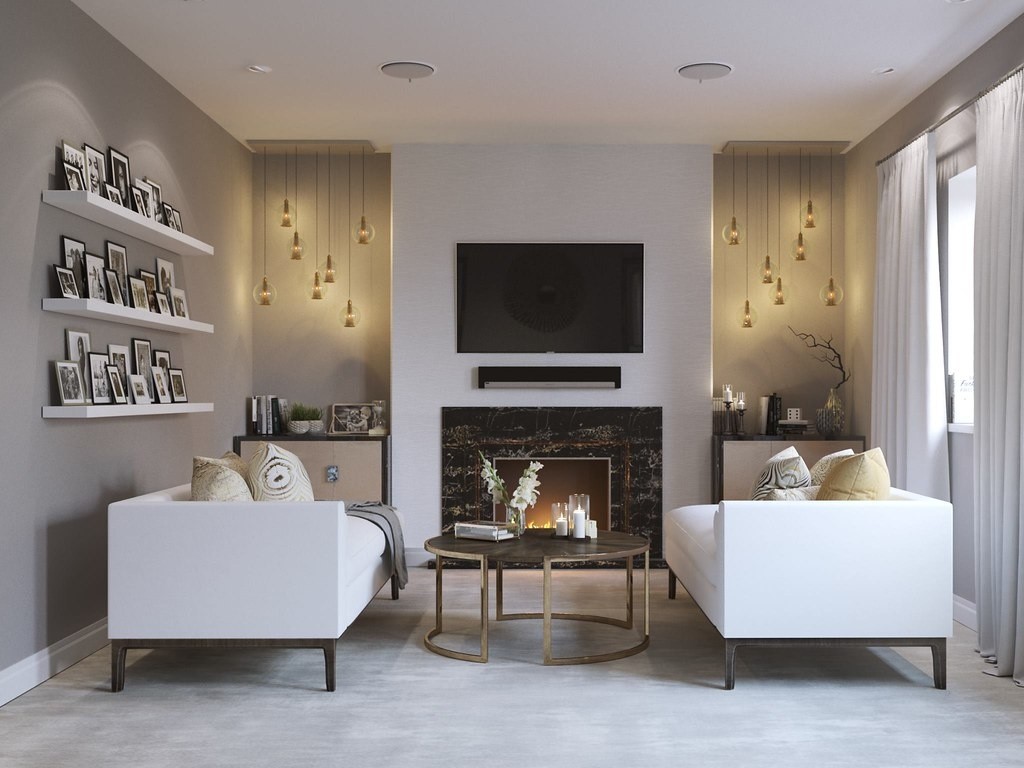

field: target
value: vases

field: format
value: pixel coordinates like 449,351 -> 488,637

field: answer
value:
505,503 -> 526,535
825,388 -> 845,437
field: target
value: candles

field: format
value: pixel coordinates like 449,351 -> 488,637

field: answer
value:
551,493 -> 591,542
737,399 -> 745,410
723,387 -> 732,401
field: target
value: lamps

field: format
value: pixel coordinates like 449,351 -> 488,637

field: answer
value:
721,141 -> 850,327
247,140 -> 375,327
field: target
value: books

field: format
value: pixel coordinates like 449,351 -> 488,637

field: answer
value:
251,395 -> 288,435
776,419 -> 819,434
758,392 -> 782,435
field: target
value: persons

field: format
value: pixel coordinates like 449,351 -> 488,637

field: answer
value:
348,406 -> 371,431
66,151 -> 181,232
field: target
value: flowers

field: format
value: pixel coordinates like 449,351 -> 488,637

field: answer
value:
478,449 -> 544,531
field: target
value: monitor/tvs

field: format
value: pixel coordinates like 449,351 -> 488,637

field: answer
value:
457,239 -> 644,353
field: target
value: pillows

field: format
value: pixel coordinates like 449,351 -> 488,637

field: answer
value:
191,440 -> 314,502
750,445 -> 890,500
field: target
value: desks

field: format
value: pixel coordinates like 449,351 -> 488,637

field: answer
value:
424,528 -> 650,666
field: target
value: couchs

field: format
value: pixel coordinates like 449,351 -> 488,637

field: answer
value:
108,484 -> 399,692
662,488 -> 953,690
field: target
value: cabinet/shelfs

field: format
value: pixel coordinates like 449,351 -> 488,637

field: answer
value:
42,189 -> 215,419
234,435 -> 391,505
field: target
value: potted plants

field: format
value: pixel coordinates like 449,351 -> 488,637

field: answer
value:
286,402 -> 325,435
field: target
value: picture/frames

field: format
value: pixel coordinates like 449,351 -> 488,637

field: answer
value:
332,404 -> 377,433
54,329 -> 189,406
60,139 -> 183,233
54,235 -> 189,320
